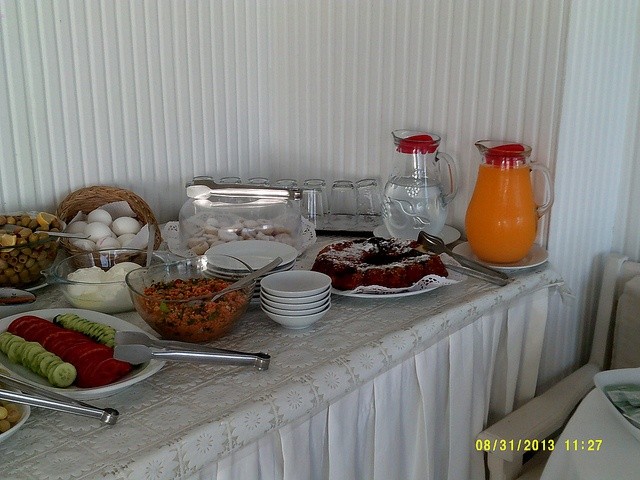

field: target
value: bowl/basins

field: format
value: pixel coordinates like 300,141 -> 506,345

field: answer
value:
259,269 -> 332,329
179,198 -> 302,254
40,248 -> 172,313
125,255 -> 257,344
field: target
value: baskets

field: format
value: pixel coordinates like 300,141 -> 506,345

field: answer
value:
57,186 -> 163,272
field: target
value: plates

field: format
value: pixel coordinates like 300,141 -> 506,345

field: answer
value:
451,241 -> 550,271
331,287 -> 440,299
0,287 -> 37,315
373,224 -> 462,246
0,387 -> 31,443
204,240 -> 298,302
0,307 -> 166,401
25,282 -> 47,292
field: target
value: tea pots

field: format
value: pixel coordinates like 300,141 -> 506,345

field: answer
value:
381,129 -> 458,240
464,140 -> 554,266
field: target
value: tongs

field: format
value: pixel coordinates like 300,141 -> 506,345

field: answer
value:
113,330 -> 271,373
0,374 -> 120,425
184,181 -> 303,200
416,230 -> 509,287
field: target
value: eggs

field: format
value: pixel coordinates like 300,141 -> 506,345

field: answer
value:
111,216 -> 140,236
68,221 -> 86,235
70,239 -> 94,251
117,234 -> 136,243
120,238 -> 135,248
96,238 -> 118,251
87,208 -> 112,225
84,221 -> 110,241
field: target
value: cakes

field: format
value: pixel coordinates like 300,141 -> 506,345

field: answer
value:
308,236 -> 449,290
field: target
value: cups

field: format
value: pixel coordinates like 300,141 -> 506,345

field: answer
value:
193,175 -> 213,180
275,178 -> 298,186
219,176 -> 242,185
301,179 -> 329,224
355,178 -> 381,227
328,179 -> 356,226
247,176 -> 271,186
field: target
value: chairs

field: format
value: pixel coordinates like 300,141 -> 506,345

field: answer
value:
476,253 -> 640,480
